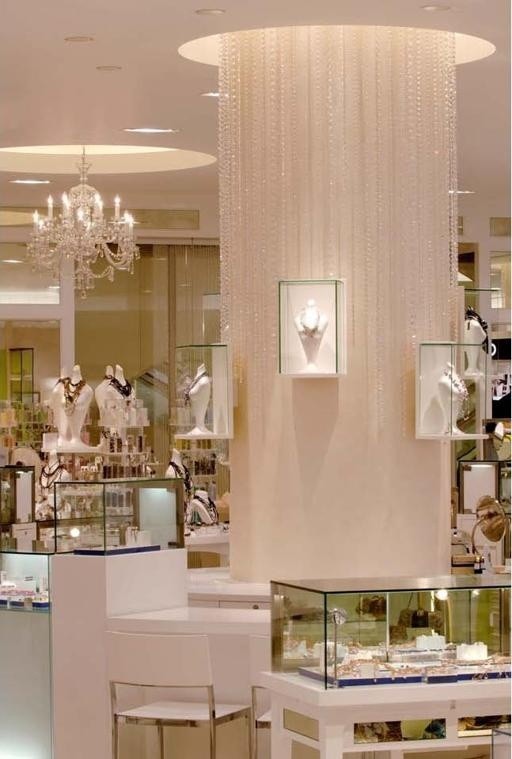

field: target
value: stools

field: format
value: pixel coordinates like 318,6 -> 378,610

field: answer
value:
109,680 -> 252,759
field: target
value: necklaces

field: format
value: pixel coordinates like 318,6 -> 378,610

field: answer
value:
444,369 -> 468,420
300,312 -> 320,337
39,372 -> 219,523
